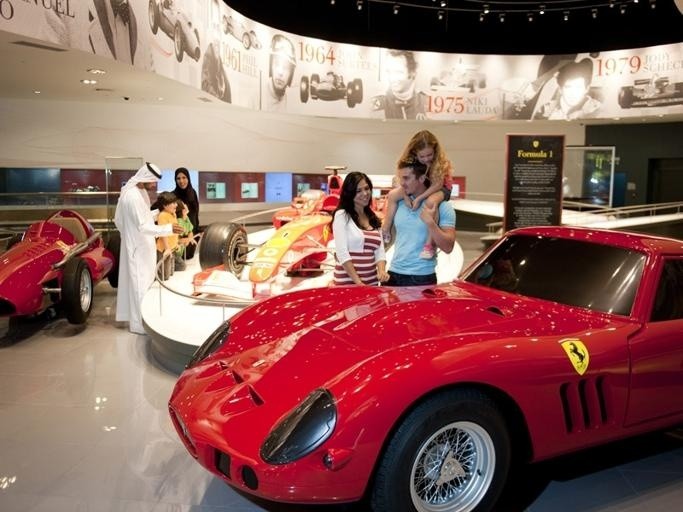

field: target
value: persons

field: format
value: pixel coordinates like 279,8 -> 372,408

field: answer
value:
268,34 -> 296,110
114,161 -> 199,335
383,50 -> 430,120
533,58 -> 602,120
330,129 -> 457,287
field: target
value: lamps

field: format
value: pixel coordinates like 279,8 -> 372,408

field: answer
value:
592,8 -> 597,18
479,13 -> 485,21
538,3 -> 545,14
393,5 -> 399,14
527,12 -> 534,21
610,1 -> 614,8
563,9 -> 569,21
441,1 -> 446,7
483,3 -> 490,13
619,4 -> 627,13
357,2 -> 363,10
331,1 -> 336,5
498,13 -> 505,22
438,11 -> 444,20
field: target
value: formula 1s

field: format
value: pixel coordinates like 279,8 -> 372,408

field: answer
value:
617,76 -> 683,109
191,166 -> 388,301
300,71 -> 363,108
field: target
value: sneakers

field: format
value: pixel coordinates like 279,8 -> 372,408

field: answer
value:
419,244 -> 436,260
382,232 -> 391,243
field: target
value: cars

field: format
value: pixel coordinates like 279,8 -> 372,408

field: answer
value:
0,209 -> 121,346
149,0 -> 200,62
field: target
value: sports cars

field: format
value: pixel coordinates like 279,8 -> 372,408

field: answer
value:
168,225 -> 683,512
